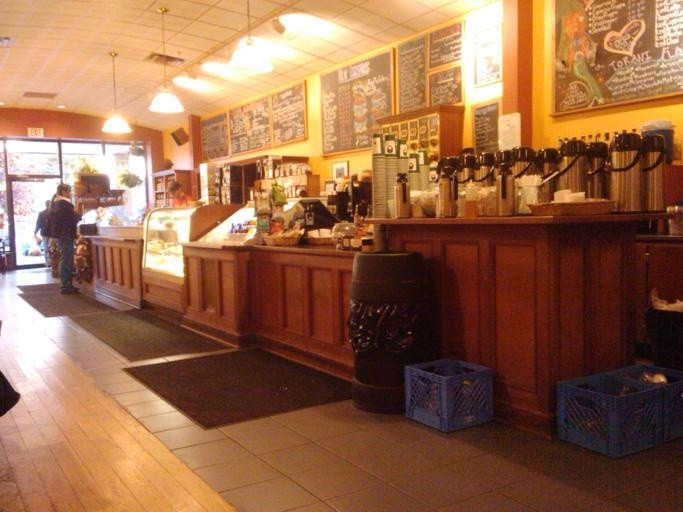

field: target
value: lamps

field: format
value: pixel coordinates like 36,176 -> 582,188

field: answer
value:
226,1 -> 273,79
271,13 -> 287,36
100,52 -> 134,138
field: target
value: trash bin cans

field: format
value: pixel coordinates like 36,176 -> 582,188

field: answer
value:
642,296 -> 683,372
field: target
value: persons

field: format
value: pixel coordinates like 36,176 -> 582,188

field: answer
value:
167,180 -> 193,208
33,200 -> 53,268
48,183 -> 82,295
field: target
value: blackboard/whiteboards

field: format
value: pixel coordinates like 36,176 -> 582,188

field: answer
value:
319,21 -> 464,158
199,80 -> 307,163
550,0 -> 683,118
470,97 -> 503,157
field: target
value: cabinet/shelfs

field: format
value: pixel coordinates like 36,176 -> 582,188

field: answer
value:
150,169 -> 198,208
255,174 -> 320,199
217,155 -> 309,204
373,103 -> 466,167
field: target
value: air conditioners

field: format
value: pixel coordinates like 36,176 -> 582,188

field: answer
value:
148,6 -> 187,117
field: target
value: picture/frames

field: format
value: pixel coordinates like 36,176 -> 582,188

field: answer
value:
330,160 -> 349,183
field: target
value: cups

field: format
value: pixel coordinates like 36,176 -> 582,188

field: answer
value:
566,192 -> 585,202
336,233 -> 372,253
370,133 -> 385,252
428,163 -> 437,192
464,187 -> 476,217
418,150 -> 427,191
665,205 -> 683,236
319,228 -> 333,238
397,139 -> 408,176
553,188 -> 571,202
306,230 -> 318,238
384,135 -> 396,218
408,152 -> 419,191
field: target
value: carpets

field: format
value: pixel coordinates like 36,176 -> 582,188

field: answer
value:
114,347 -> 353,431
15,281 -> 121,318
68,307 -> 233,363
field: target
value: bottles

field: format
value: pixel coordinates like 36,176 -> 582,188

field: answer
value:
396,173 -> 410,219
558,127 -> 637,169
559,12 -> 602,110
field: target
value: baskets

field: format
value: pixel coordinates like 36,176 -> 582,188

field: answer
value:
403,358 -> 494,433
556,363 -> 682,459
261,233 -> 301,246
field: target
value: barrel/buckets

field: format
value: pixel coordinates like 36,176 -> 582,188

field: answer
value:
346,250 -> 437,415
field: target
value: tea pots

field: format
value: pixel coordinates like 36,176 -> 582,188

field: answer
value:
517,185 -> 543,216
331,220 -> 358,246
